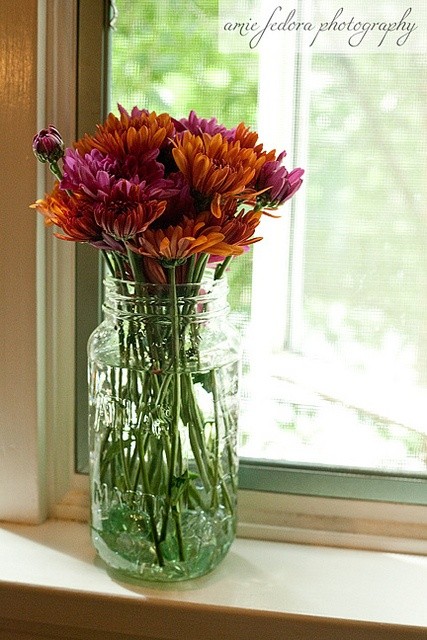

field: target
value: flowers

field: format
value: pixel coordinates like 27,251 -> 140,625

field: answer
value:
83,266 -> 244,591
25,100 -> 309,565
